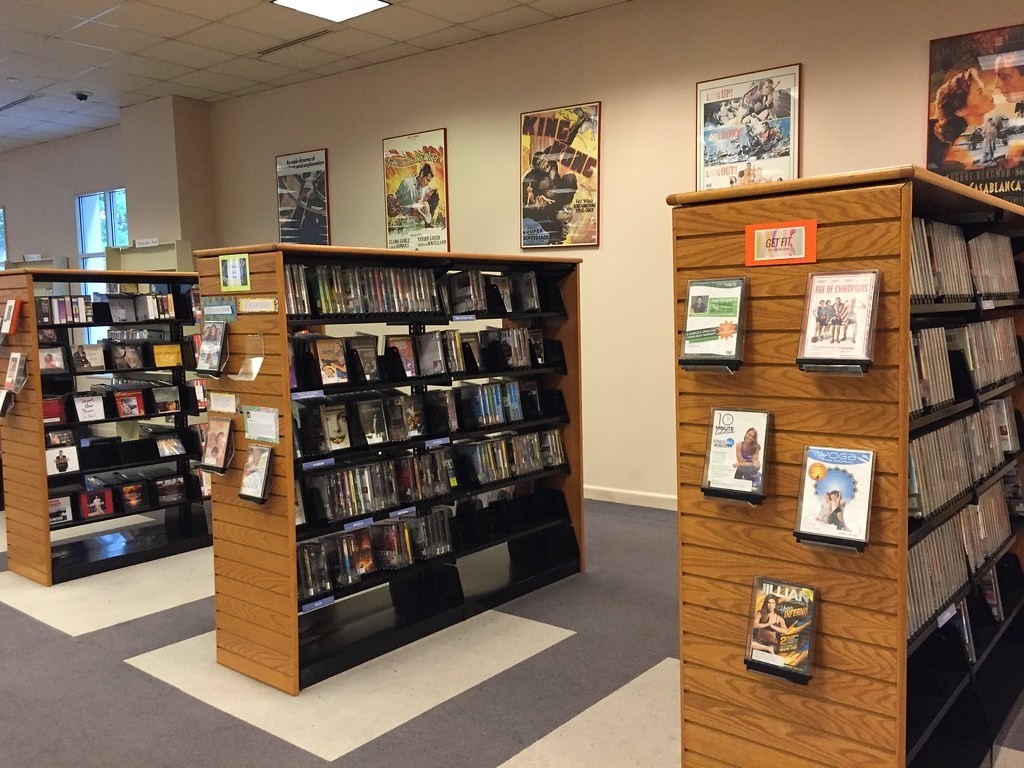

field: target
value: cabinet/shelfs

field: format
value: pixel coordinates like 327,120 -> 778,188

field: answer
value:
0,268 -> 213,588
666,165 -> 1023,768
4,256 -> 69,297
194,243 -> 587,697
105,240 -> 194,296
